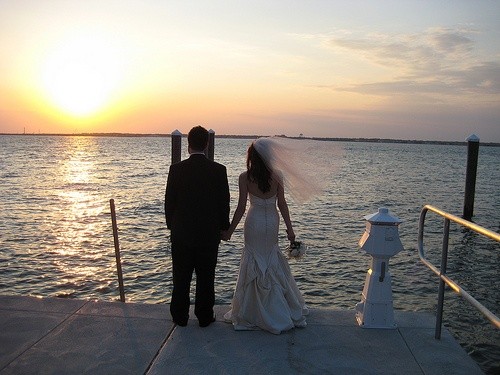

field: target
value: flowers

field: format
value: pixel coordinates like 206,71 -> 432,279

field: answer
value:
286,230 -> 306,260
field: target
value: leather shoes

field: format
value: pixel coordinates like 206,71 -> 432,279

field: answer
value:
173,317 -> 188,327
198,317 -> 216,327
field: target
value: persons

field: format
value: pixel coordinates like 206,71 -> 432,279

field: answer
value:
165,125 -> 232,327
223,137 -> 296,335
